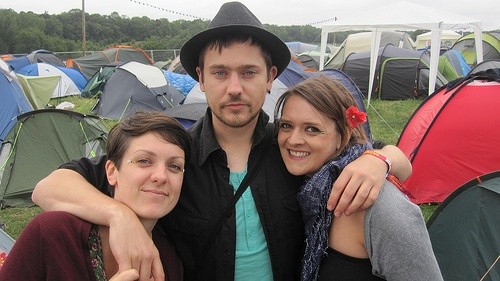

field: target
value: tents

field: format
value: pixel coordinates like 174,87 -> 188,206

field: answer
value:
318,0 -> 483,109
0,30 -> 500,281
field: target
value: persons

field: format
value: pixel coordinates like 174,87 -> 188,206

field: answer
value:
0,111 -> 192,281
31,2 -> 413,281
273,75 -> 443,281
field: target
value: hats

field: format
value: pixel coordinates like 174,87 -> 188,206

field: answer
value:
180,1 -> 292,83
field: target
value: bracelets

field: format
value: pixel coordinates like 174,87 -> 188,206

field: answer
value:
362,150 -> 392,174
